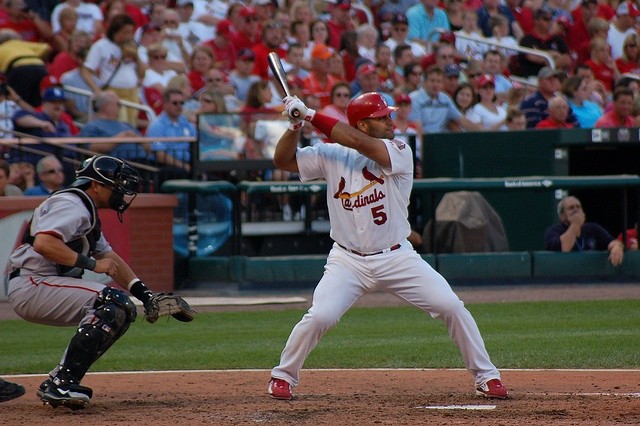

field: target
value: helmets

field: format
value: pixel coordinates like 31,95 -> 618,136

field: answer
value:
347,93 -> 399,126
71,156 -> 140,223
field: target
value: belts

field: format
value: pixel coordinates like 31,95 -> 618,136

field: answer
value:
338,243 -> 401,257
9,267 -> 19,280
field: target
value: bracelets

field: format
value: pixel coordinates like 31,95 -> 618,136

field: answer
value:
76,254 -> 96,270
311,113 -> 339,138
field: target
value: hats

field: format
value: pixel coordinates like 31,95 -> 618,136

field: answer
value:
240,8 -> 256,17
393,14 -> 408,25
238,49 -> 254,61
538,66 -> 568,79
360,65 -> 377,74
45,88 -> 65,102
444,64 -> 459,77
312,45 -> 334,60
480,75 -> 495,86
216,20 -> 238,40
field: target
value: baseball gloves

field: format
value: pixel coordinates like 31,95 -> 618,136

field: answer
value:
144,292 -> 198,324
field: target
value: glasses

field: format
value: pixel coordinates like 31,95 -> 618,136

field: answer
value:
208,78 -> 221,82
47,169 -> 65,172
568,205 -> 581,209
153,55 -> 166,59
205,99 -> 214,103
172,101 -> 184,105
411,72 -> 423,76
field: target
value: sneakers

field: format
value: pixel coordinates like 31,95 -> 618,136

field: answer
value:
476,379 -> 507,399
41,377 -> 92,411
37,379 -> 53,395
268,377 -> 292,399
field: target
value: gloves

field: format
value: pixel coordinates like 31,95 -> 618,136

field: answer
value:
282,96 -> 316,131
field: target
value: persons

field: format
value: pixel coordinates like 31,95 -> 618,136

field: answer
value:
391,1 -> 639,179
545,196 -> 623,267
4,155 -> 196,411
293,1 -> 390,219
270,91 -> 508,400
1,0 -> 292,221
619,228 -> 638,248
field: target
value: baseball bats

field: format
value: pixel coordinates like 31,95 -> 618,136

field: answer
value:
267,52 -> 300,118
